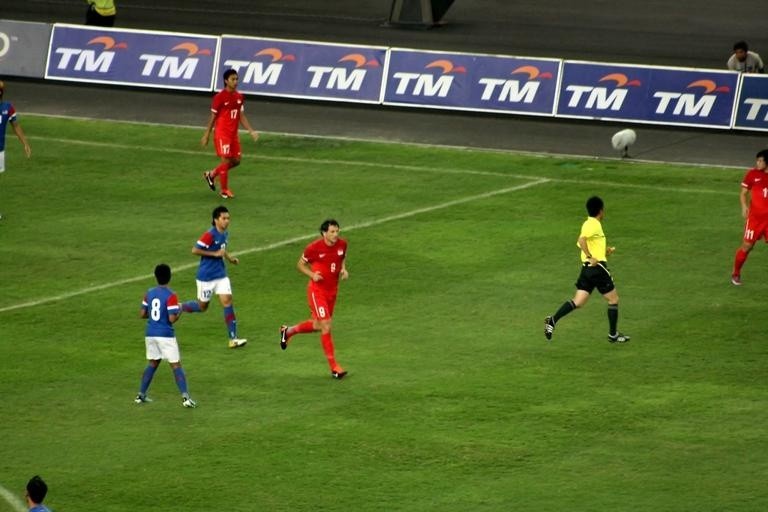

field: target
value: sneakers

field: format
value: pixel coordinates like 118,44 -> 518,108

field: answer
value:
331,365 -> 348,380
280,324 -> 289,350
182,397 -> 199,408
228,337 -> 247,349
134,393 -> 154,404
608,331 -> 630,344
201,171 -> 217,192
544,316 -> 555,341
218,189 -> 237,200
731,273 -> 743,289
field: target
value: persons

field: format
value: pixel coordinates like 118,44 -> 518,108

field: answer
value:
176,206 -> 247,348
726,41 -> 764,74
133,264 -> 199,408
279,219 -> 348,379
26,476 -> 55,512
544,196 -> 631,342
731,150 -> 768,286
201,69 -> 261,199
0,80 -> 31,173
85,0 -> 116,27
